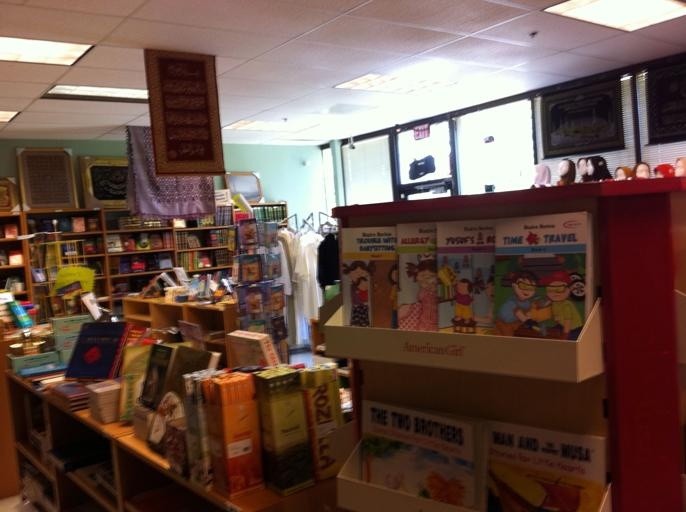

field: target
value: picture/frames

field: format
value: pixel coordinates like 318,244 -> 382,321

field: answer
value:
0,146 -> 131,213
222,170 -> 266,205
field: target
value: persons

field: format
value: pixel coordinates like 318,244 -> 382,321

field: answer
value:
529,155 -> 686,190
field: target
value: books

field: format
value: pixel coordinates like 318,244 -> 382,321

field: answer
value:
337,209 -> 606,512
30,207 -> 286,460
